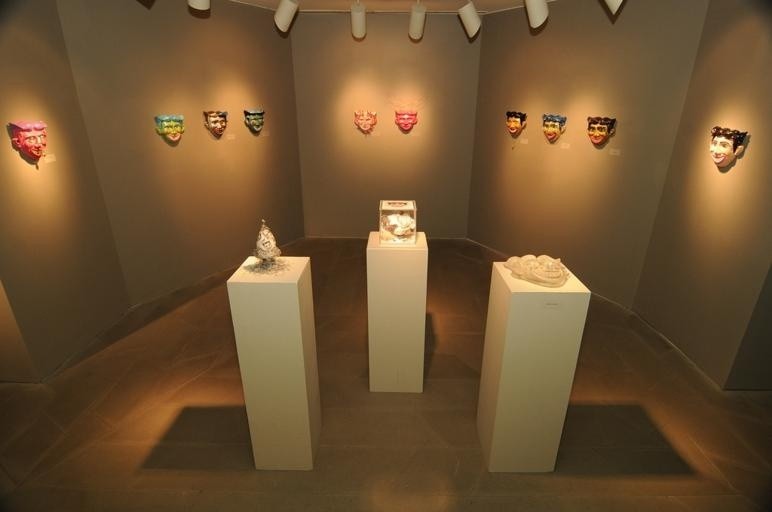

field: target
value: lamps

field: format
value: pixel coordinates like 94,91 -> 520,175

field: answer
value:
187,1 -> 211,12
603,0 -> 625,16
523,0 -> 551,34
272,0 -> 298,34
408,0 -> 429,41
458,1 -> 483,39
349,0 -> 370,39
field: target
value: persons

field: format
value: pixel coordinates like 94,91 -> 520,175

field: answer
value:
708,126 -> 748,169
202,110 -> 228,138
154,113 -> 185,146
586,116 -> 617,145
543,113 -> 566,144
506,111 -> 526,136
394,110 -> 418,132
8,120 -> 48,160
353,111 -> 377,133
243,110 -> 265,133
